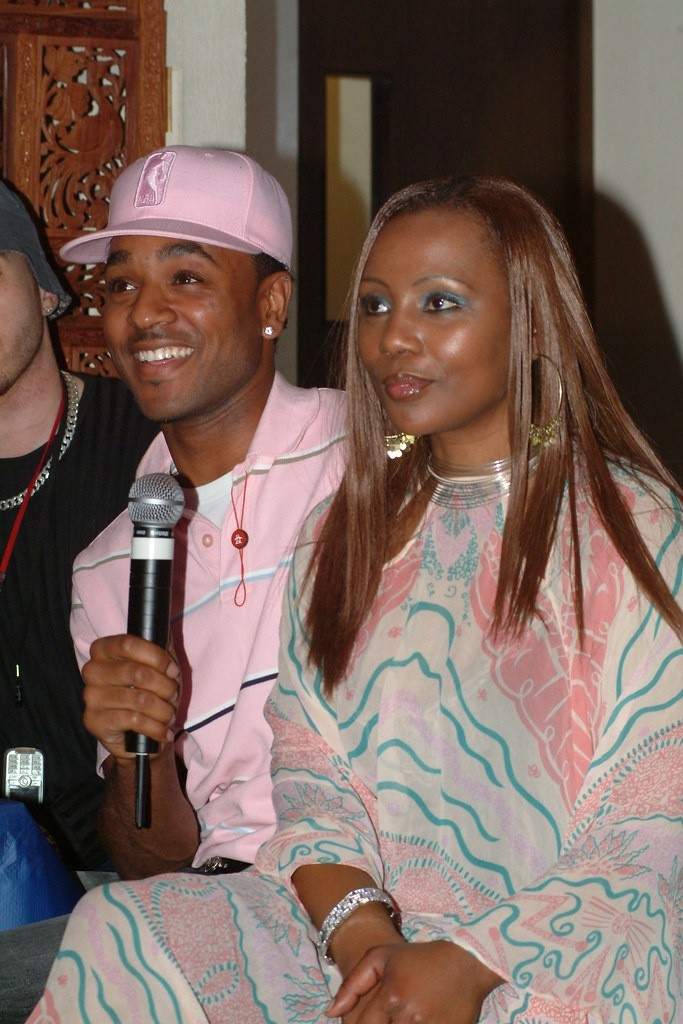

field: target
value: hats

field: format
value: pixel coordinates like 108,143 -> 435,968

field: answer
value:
57,144 -> 294,279
0,178 -> 72,320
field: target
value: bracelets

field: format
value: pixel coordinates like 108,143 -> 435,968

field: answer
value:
317,887 -> 398,966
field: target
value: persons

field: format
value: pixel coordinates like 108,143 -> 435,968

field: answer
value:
25,177 -> 683,1024
0,144 -> 347,1024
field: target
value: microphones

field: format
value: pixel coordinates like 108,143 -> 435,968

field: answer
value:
127,471 -> 185,831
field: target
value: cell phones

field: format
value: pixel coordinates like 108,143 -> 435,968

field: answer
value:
5,748 -> 44,805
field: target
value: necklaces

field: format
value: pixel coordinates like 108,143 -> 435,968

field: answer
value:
418,446 -> 539,509
0,369 -> 78,511
